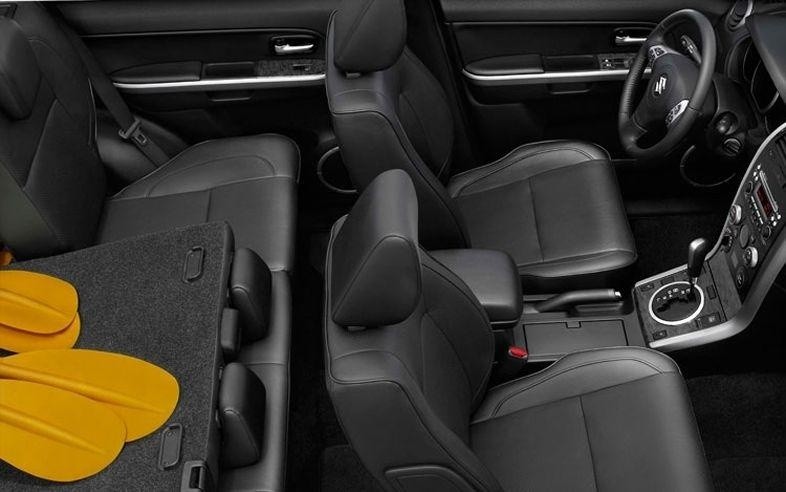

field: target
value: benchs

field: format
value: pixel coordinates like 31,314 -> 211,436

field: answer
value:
1,0 -> 322,491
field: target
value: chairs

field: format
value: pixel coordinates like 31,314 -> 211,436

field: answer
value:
324,170 -> 714,492
327,3 -> 640,295
